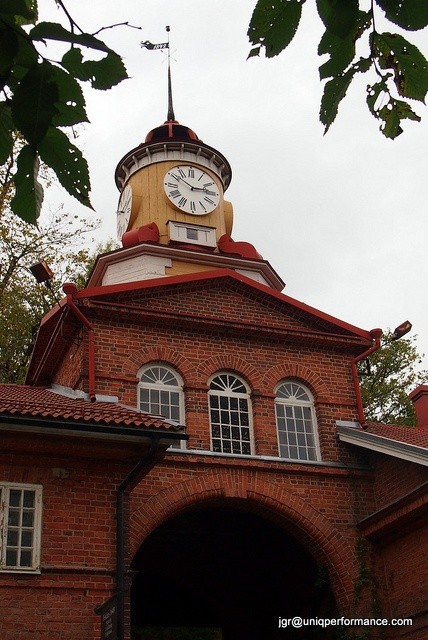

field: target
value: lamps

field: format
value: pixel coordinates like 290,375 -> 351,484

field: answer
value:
383,320 -> 412,346
28,260 -> 69,325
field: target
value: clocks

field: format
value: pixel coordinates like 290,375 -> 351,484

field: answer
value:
163,165 -> 221,216
116,184 -> 133,242
